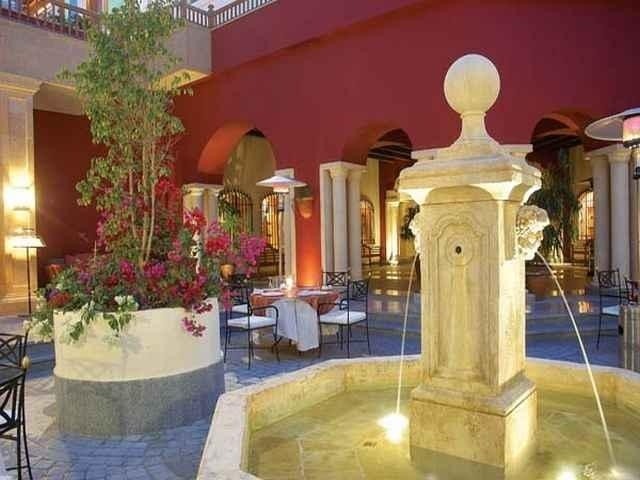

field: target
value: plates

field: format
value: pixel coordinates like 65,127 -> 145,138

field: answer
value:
262,292 -> 282,297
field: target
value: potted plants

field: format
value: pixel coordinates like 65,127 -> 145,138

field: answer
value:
526,142 -> 581,296
401,204 -> 420,280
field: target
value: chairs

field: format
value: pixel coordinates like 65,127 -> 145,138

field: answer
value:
257,242 -> 285,277
326,271 -> 353,339
224,286 -> 280,370
361,238 -> 381,266
226,274 -> 262,344
596,268 -> 629,349
316,273 -> 372,359
624,276 -> 640,306
0,328 -> 34,480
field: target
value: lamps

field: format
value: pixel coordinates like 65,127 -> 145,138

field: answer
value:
256,175 -> 307,284
9,227 -> 46,317
584,105 -> 640,279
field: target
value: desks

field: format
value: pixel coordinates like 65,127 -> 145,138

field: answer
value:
251,288 -> 339,353
